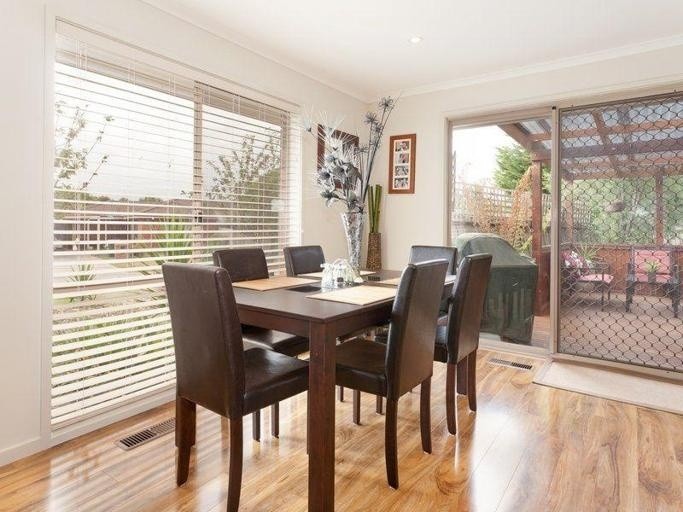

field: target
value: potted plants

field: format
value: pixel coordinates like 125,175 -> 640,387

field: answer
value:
574,243 -> 600,291
642,260 -> 661,286
290,88 -> 409,276
366,184 -> 382,270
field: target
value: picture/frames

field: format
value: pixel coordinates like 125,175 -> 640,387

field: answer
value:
388,134 -> 416,195
317,124 -> 360,191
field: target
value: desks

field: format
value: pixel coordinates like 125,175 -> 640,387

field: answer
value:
174,269 -> 458,512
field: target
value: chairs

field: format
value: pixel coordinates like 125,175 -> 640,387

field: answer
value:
546,243 -> 615,313
283,245 -> 371,401
373,245 -> 458,394
335,258 -> 449,490
212,246 -> 309,442
161,262 -> 309,511
374,253 -> 493,436
625,242 -> 681,318
